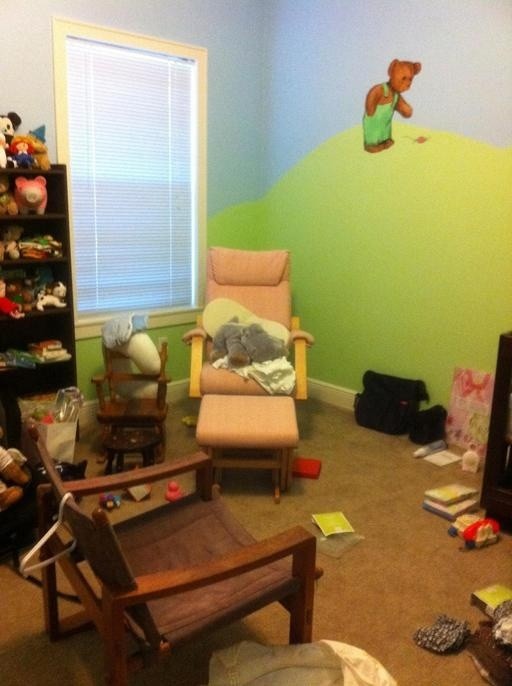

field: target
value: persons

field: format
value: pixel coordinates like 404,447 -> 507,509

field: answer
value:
0,443 -> 32,513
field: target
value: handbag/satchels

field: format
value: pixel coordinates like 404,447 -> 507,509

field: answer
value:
354,370 -> 428,435
409,405 -> 446,445
21,400 -> 77,470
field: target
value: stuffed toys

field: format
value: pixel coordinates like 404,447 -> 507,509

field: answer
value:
0,110 -> 67,321
200,296 -> 291,352
208,313 -> 287,368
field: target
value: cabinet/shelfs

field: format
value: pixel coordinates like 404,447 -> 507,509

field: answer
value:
482,331 -> 512,535
1,163 -> 80,443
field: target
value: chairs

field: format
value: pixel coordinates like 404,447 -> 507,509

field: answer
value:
184,247 -> 315,416
91,341 -> 171,464
31,441 -> 324,686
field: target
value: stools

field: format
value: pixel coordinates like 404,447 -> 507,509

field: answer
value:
197,393 -> 299,504
98,431 -> 160,475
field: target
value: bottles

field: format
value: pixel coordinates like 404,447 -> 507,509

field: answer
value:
462,443 -> 481,473
412,439 -> 447,459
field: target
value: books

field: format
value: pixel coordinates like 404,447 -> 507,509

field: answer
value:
423,482 -> 480,507
0,338 -> 73,369
470,584 -> 512,618
423,497 -> 481,520
311,509 -> 355,538
